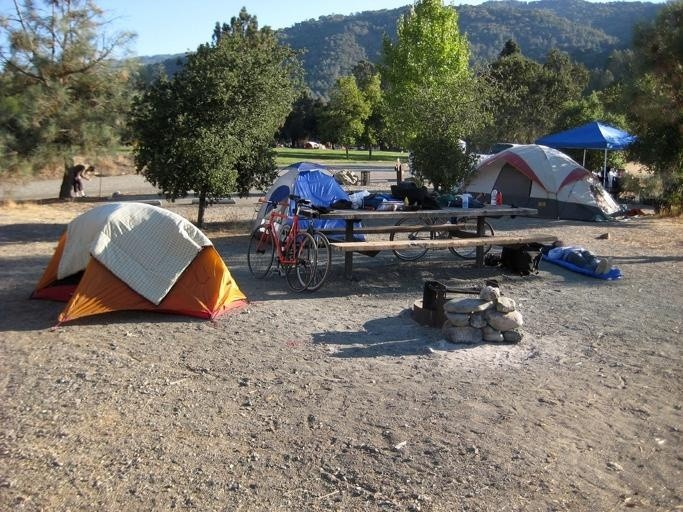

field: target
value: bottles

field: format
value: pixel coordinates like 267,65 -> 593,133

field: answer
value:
496,191 -> 502,207
491,188 -> 497,206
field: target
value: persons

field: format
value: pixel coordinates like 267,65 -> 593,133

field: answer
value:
592,165 -> 625,190
346,190 -> 409,211
547,240 -> 613,276
69,162 -> 96,198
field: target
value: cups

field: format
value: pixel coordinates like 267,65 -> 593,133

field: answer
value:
462,195 -> 468,209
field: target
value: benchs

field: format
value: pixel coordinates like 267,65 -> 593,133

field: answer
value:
299,223 -> 465,234
330,234 -> 558,280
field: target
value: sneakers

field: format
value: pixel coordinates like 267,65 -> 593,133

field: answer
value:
603,257 -> 613,274
593,258 -> 608,275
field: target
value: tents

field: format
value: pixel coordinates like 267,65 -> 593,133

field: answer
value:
252,160 -> 365,243
456,142 -> 628,223
30,201 -> 250,331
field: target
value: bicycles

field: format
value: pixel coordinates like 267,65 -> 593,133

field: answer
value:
282,194 -> 331,291
389,200 -> 494,262
247,196 -> 317,294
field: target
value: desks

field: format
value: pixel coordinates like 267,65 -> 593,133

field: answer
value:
320,208 -> 539,240
